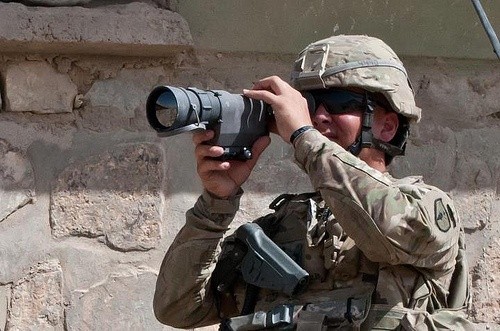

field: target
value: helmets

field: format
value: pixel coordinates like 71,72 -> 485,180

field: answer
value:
292,34 -> 421,120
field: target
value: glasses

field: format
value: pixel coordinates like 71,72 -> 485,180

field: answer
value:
302,89 -> 388,115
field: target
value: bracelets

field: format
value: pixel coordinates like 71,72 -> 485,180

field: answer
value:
290,126 -> 315,145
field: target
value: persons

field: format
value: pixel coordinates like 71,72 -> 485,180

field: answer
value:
153,35 -> 471,331
